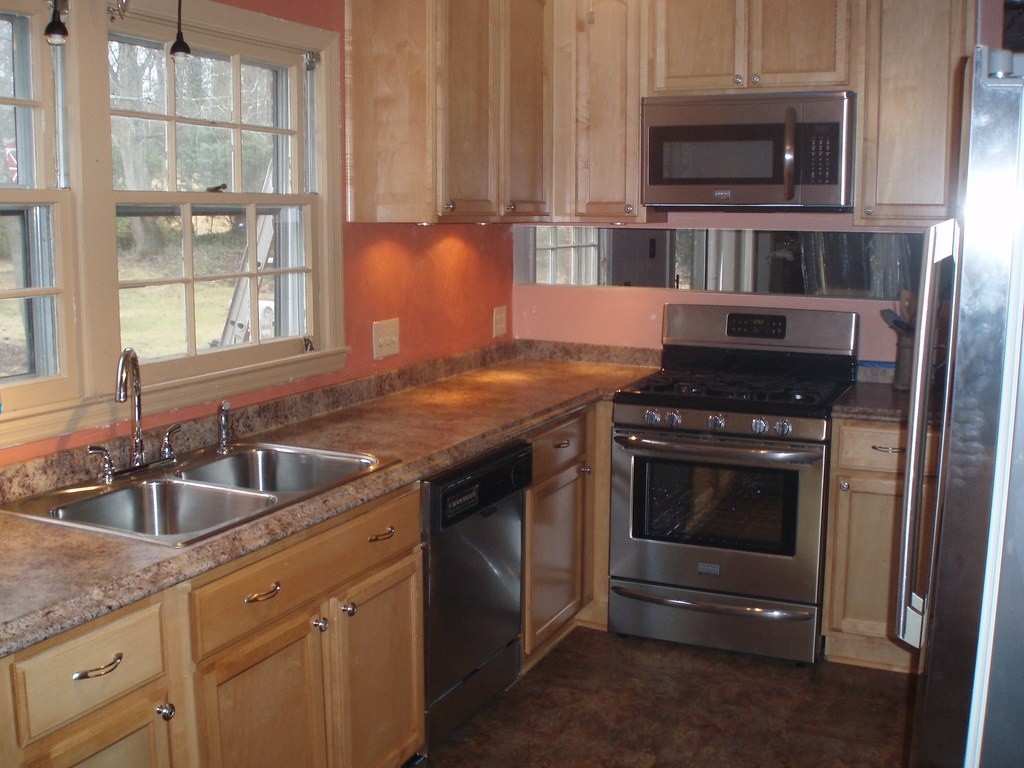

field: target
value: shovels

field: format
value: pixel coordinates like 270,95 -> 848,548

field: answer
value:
900,289 -> 917,324
881,309 -> 900,328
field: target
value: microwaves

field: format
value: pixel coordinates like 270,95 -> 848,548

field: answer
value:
638,89 -> 858,212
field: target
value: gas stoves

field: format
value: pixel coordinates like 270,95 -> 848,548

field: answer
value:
612,303 -> 860,420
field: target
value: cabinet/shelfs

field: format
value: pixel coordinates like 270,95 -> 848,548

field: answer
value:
522,400 -> 595,676
850,0 -> 976,226
1,480 -> 425,768
822,415 -> 945,676
644,0 -> 850,93
545,0 -> 644,227
344,0 -> 552,223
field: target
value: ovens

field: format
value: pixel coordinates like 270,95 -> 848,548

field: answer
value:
609,403 -> 827,666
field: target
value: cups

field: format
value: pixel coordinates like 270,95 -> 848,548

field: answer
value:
893,334 -> 915,391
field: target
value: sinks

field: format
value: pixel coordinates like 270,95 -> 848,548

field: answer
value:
142,442 -> 400,500
0,469 -> 296,546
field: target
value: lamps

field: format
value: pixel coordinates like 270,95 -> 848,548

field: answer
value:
169,0 -> 190,60
44,0 -> 69,46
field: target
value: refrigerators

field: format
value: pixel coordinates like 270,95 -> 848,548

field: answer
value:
892,43 -> 1024,768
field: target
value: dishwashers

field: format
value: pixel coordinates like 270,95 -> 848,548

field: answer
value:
419,439 -> 526,759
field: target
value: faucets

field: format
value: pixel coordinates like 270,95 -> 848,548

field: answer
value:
215,399 -> 232,455
87,347 -> 182,485
